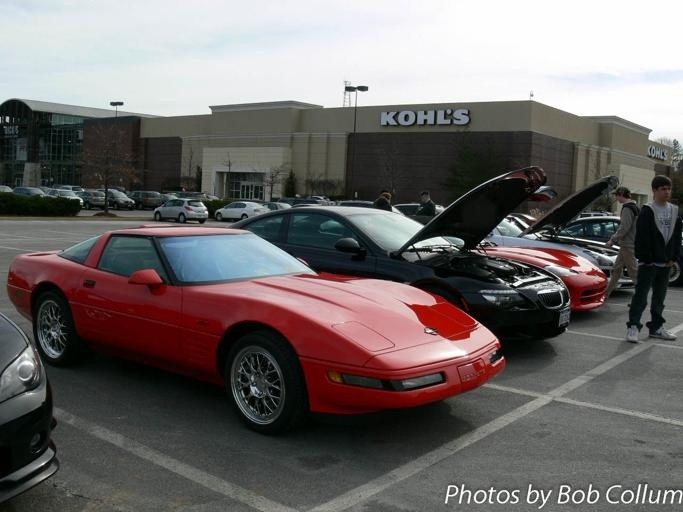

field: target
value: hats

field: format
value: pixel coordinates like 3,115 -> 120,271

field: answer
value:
610,187 -> 631,197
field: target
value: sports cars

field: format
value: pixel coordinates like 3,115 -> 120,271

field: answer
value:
440,230 -> 611,314
226,166 -> 573,349
4,221 -> 509,437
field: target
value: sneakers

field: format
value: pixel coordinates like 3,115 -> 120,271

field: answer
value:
626,325 -> 639,342
649,325 -> 677,339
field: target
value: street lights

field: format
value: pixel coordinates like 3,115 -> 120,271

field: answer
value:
106,99 -> 123,121
343,83 -> 368,194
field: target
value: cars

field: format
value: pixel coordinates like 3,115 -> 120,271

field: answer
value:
0,311 -> 61,504
151,197 -> 210,224
212,200 -> 271,221
0,182 -> 224,209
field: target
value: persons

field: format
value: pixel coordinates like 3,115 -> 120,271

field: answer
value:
366,189 -> 393,212
625,174 -> 683,343
604,186 -> 640,307
416,190 -> 435,216
